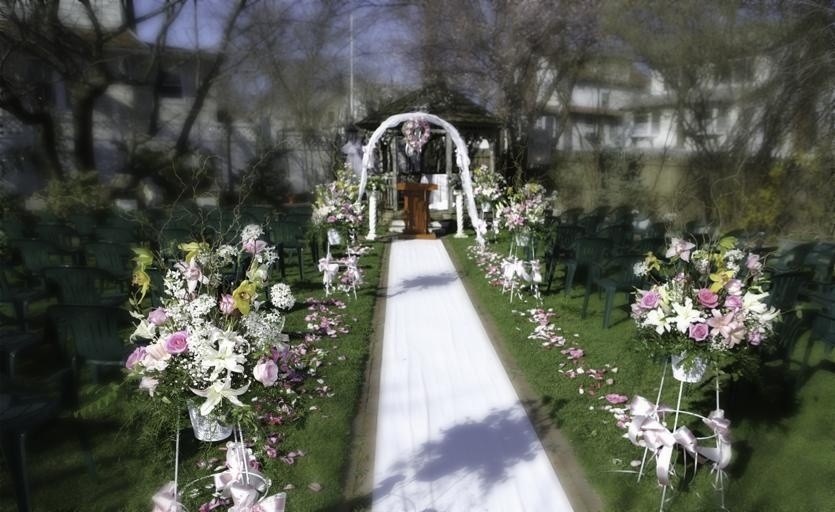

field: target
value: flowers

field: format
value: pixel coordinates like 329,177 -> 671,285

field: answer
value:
469,163 -> 563,239
115,225 -> 297,442
402,119 -> 431,150
308,163 -> 365,229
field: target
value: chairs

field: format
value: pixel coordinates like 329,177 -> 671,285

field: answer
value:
545,205 -> 834,385
0,207 -> 325,512
627,227 -> 785,393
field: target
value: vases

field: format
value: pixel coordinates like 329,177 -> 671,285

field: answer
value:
515,226 -> 531,248
186,393 -> 234,442
327,227 -> 342,245
